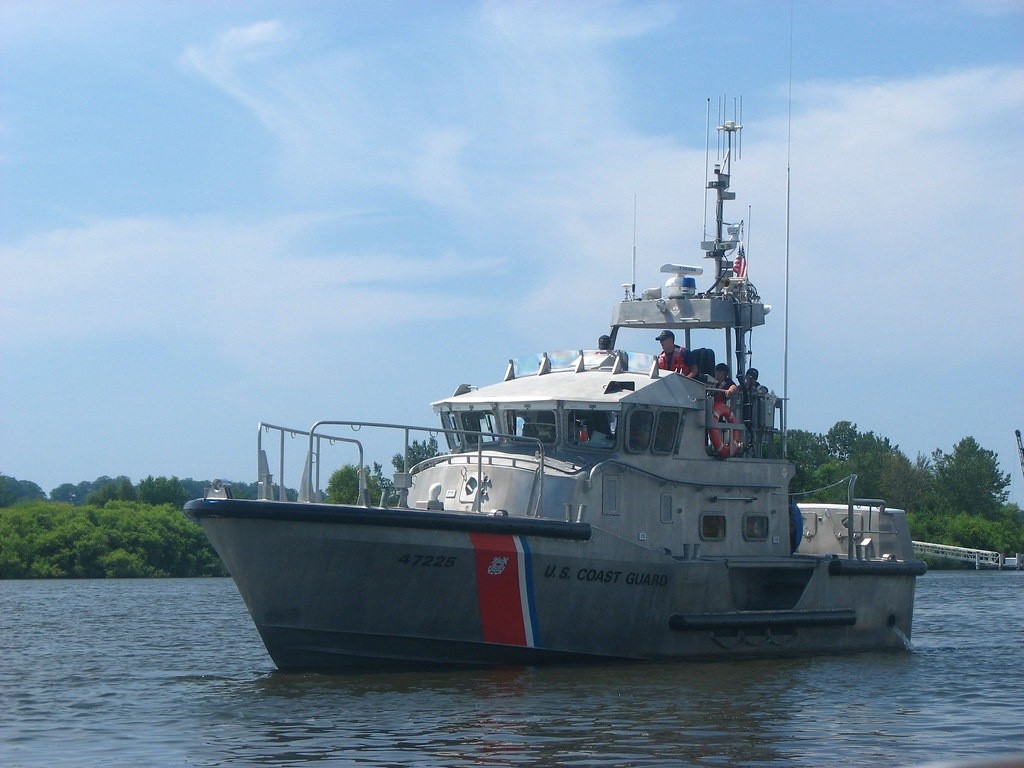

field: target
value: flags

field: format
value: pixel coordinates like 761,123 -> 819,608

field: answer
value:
733,242 -> 747,278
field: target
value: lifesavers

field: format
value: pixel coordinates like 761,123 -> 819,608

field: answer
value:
709,403 -> 739,457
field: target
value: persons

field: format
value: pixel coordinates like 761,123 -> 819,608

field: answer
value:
713,362 -> 738,409
654,330 -> 698,380
598,335 -> 611,350
739,368 -> 768,421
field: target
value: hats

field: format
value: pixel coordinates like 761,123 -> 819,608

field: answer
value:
745,368 -> 758,379
655,330 -> 674,341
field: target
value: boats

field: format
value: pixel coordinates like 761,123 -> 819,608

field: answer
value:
181,93 -> 928,674
